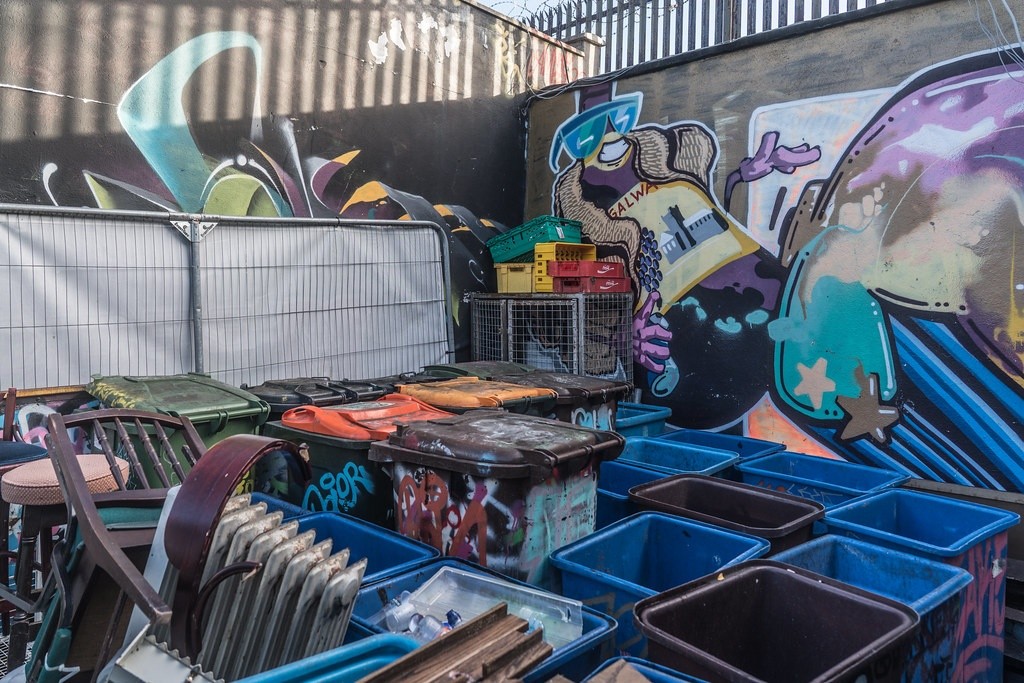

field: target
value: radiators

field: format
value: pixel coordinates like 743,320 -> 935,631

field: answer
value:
146,493 -> 370,683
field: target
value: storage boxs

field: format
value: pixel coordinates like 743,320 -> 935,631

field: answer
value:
483,211 -> 630,294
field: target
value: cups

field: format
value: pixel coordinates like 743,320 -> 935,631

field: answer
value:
384,595 -> 463,646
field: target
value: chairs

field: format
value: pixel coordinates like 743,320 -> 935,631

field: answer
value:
24,409 -> 209,683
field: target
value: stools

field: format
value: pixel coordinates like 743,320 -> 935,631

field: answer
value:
1,451 -> 130,672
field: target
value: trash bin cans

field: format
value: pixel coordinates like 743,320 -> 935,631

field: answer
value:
89,360 -> 998,681
